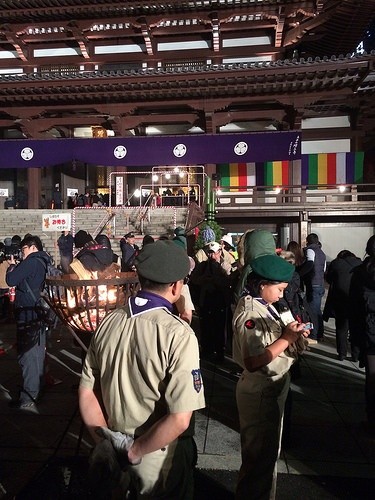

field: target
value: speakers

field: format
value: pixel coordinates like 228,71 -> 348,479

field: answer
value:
212,173 -> 221,180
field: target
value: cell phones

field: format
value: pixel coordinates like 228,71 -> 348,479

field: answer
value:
303,323 -> 314,331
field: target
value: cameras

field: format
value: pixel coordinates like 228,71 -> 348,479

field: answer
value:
5,251 -> 19,259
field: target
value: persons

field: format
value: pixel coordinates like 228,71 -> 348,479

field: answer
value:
141,186 -> 197,206
4,198 -> 10,209
6,234 -> 51,407
15,198 -> 21,208
323,249 -> 364,362
41,196 -> 47,209
67,189 -> 116,210
231,254 -> 310,500
349,235 -> 375,432
77,240 -> 206,500
0,228 -> 326,346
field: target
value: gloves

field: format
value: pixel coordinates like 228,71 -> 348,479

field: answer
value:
91,438 -> 123,468
288,333 -> 310,354
95,427 -> 141,466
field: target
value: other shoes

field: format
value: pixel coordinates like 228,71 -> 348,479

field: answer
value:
307,337 -> 318,345
339,355 -> 346,360
8,398 -> 39,407
352,358 -> 358,362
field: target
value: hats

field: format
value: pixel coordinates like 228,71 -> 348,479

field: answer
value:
250,255 -> 295,283
220,234 -> 234,247
134,240 -> 191,283
74,230 -> 93,248
205,241 -> 223,253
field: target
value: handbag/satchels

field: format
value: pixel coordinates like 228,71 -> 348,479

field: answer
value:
35,297 -> 57,324
